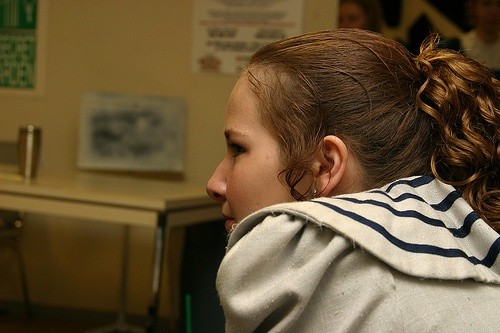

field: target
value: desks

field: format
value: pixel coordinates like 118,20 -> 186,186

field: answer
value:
0,165 -> 227,333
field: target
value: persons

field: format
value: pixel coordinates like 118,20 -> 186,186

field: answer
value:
339,0 -> 380,33
203,31 -> 500,333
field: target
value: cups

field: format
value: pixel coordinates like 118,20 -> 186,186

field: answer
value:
17,125 -> 41,178
